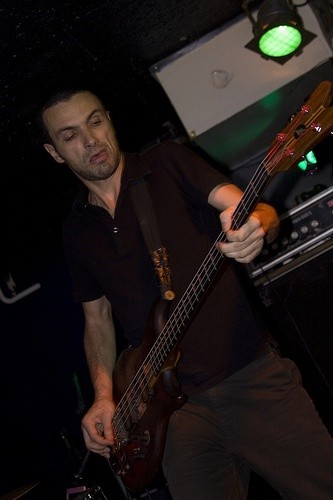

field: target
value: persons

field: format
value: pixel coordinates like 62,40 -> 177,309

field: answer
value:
31,68 -> 333,500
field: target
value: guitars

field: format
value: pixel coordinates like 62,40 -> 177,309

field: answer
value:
108,79 -> 332,487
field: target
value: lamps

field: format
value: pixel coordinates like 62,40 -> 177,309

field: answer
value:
240,1 -> 319,65
